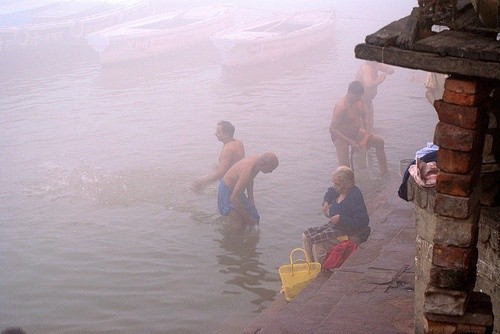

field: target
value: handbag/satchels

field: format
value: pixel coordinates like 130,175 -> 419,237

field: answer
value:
279,248 -> 322,302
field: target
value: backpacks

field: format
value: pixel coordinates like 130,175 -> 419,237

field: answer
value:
321,240 -> 357,272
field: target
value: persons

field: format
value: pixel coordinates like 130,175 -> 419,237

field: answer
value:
218,152 -> 279,237
424,72 -> 450,106
354,60 -> 394,132
302,165 -> 369,262
192,119 -> 246,195
329,81 -> 388,172
